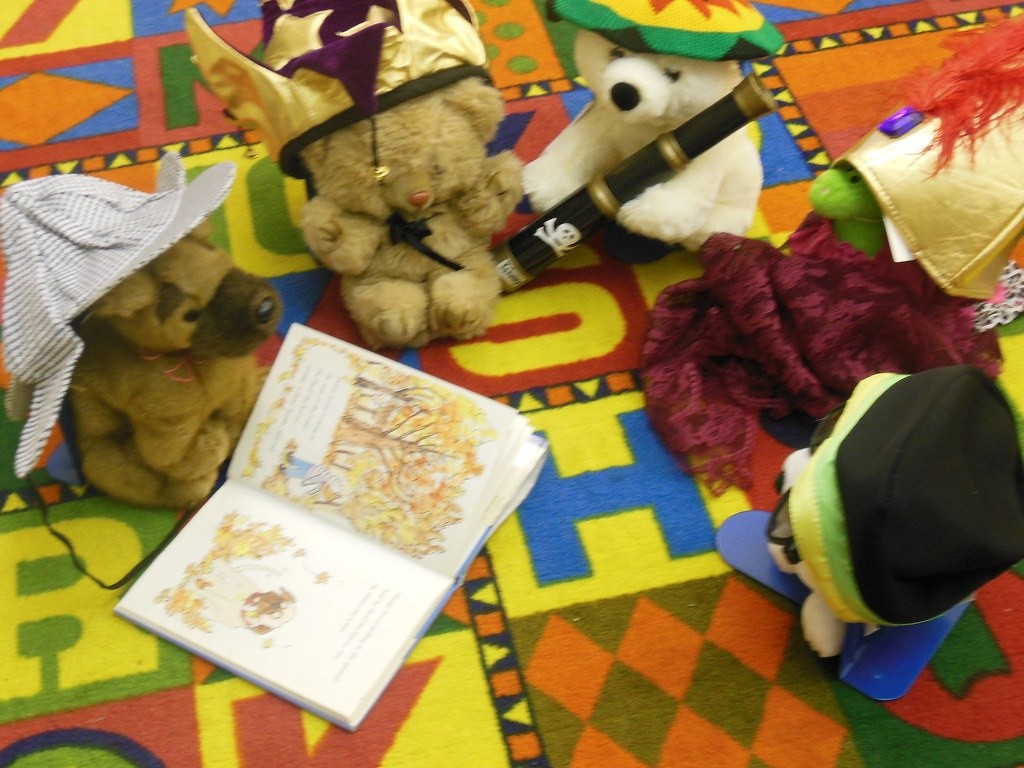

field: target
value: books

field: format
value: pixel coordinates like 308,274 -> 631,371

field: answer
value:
111,322 -> 550,732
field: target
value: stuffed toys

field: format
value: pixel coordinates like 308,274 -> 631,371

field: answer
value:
763,367 -> 1024,661
296,75 -> 526,349
521,30 -> 765,252
811,163 -> 886,261
63,218 -> 284,513
